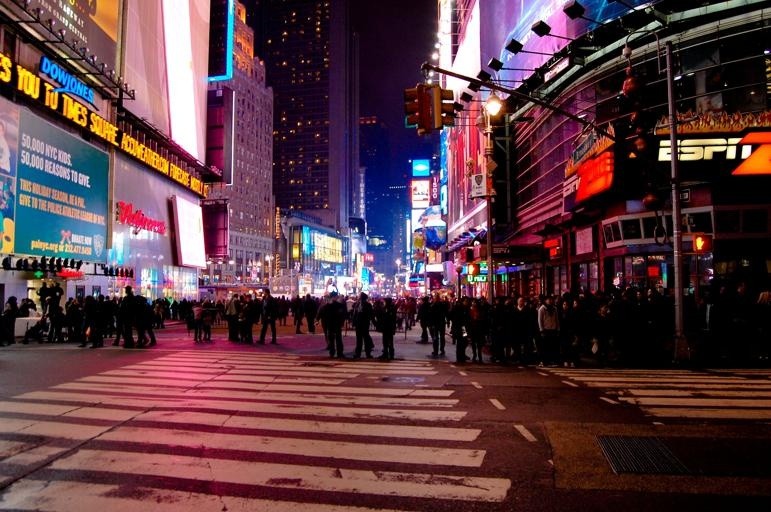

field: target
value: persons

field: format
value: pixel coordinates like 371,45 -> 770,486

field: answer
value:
2,280 -> 771,368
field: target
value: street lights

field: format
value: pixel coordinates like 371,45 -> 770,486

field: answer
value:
396,258 -> 401,280
265,254 -> 275,289
475,96 -> 508,306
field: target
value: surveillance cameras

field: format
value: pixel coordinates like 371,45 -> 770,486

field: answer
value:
622,47 -> 632,59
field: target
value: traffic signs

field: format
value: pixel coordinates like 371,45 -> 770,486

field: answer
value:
679,189 -> 690,202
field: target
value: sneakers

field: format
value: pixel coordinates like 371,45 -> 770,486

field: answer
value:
279,321 -> 316,335
189,333 -> 277,346
0,324 -> 167,349
416,340 -> 428,343
322,343 -> 396,362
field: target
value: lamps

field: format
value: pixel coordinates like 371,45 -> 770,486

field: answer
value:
440,0 -> 639,130
1,248 -> 136,279
1,1 -> 138,105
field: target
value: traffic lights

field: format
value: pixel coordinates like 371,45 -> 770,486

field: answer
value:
692,233 -> 714,254
430,83 -> 455,129
469,265 -> 480,274
630,149 -> 667,212
403,82 -> 432,136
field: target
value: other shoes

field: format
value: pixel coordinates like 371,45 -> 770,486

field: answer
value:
427,348 -> 576,369
